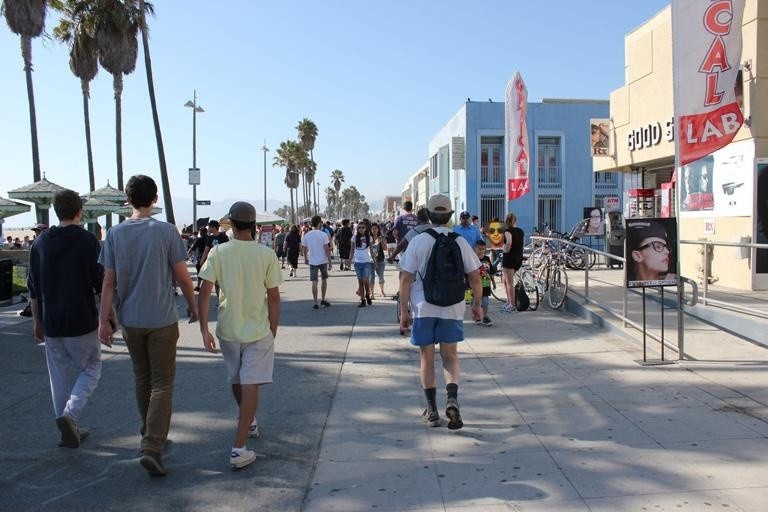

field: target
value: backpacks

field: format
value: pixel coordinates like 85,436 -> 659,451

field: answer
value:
418,228 -> 465,306
515,282 -> 529,311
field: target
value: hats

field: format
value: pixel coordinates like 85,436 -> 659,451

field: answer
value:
427,194 -> 451,214
31,224 -> 48,230
461,212 -> 469,216
628,221 -> 667,240
229,202 -> 256,222
207,220 -> 219,226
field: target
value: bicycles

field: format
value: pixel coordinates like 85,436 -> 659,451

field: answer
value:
513,230 -> 575,314
529,216 -> 594,272
478,252 -> 538,313
393,295 -> 421,330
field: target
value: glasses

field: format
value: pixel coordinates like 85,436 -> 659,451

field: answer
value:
723,183 -> 744,194
358,227 -> 365,228
488,228 -> 504,233
462,217 -> 469,219
638,241 -> 670,252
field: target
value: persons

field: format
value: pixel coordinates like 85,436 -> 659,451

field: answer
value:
454,212 -> 524,311
681,164 -> 699,210
197,200 -> 285,470
591,124 -> 609,148
98,174 -> 200,477
13,238 -> 23,248
397,193 -> 484,430
22,236 -> 31,248
470,239 -> 497,328
3,236 -> 17,249
393,200 -> 420,246
26,188 -> 117,450
583,208 -> 604,235
181,220 -> 233,306
17,223 -> 48,318
628,221 -> 676,281
255,216 -> 393,310
695,161 -> 710,208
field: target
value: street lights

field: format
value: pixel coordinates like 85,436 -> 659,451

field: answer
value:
184,90 -> 203,234
260,137 -> 269,212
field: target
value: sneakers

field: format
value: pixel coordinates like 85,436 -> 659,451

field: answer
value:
141,453 -> 167,475
422,408 -> 440,426
289,268 -> 293,276
312,305 -> 319,310
230,446 -> 256,468
367,296 -> 371,305
446,398 -> 463,429
57,416 -> 89,448
248,416 -> 260,437
320,301 -> 329,306
358,300 -> 366,307
476,317 -> 492,325
500,302 -> 517,313
341,263 -> 351,271
